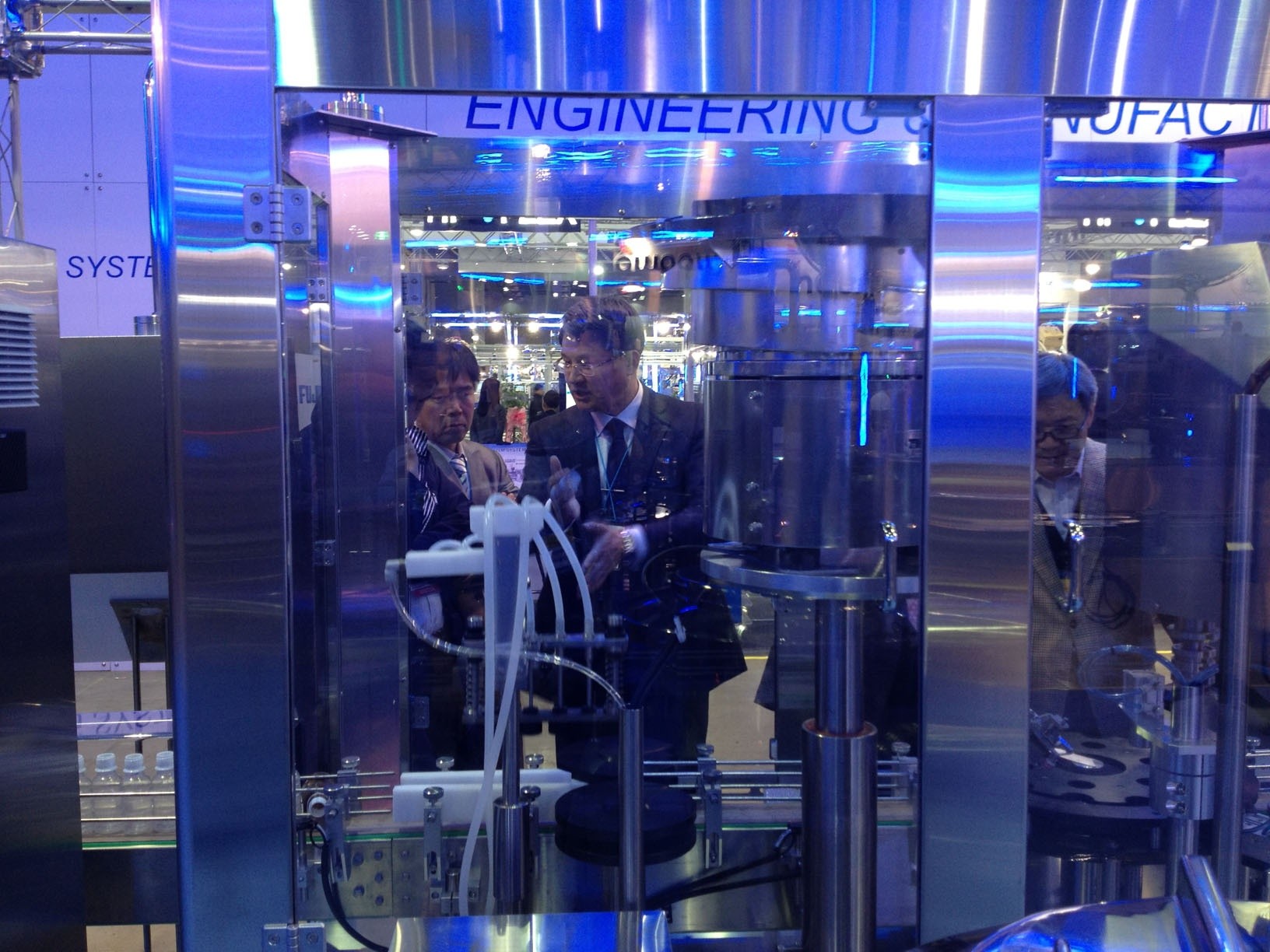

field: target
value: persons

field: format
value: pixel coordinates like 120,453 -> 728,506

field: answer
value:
292,327 -> 484,775
1029,353 -> 1158,897
1066,322 -> 1113,445
406,340 -> 516,773
743,545 -> 919,770
514,294 -> 748,783
471,372 -> 560,445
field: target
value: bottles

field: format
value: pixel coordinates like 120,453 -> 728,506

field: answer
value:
120,753 -> 152,835
293,769 -> 303,814
78,754 -> 92,836
92,752 -> 121,834
151,750 -> 175,833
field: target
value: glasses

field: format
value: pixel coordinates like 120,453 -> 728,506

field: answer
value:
1036,412 -> 1088,442
554,352 -> 624,378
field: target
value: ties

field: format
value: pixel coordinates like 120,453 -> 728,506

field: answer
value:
448,456 -> 471,499
605,419 -> 631,502
